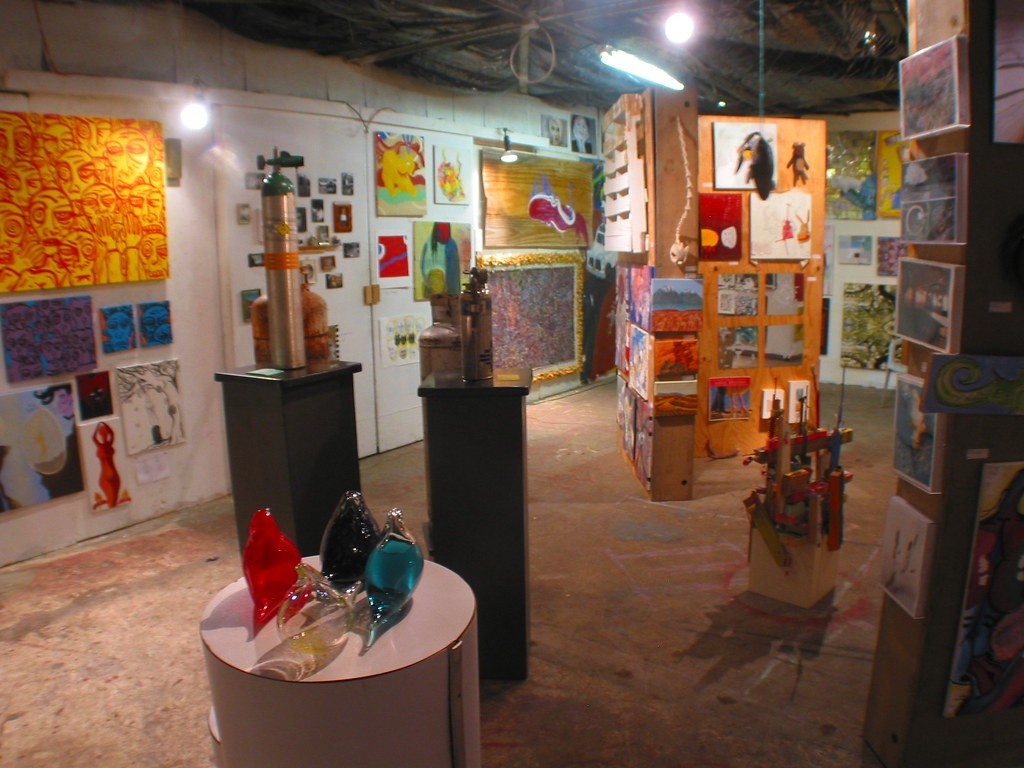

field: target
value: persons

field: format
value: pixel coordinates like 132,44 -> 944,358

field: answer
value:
547,117 -> 563,145
571,115 -> 592,153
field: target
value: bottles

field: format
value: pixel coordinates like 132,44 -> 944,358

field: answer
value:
459,269 -> 496,382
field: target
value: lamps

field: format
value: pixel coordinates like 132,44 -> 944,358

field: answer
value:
500,134 -> 517,163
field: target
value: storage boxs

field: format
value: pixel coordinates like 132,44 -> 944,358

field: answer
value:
747,527 -> 842,611
